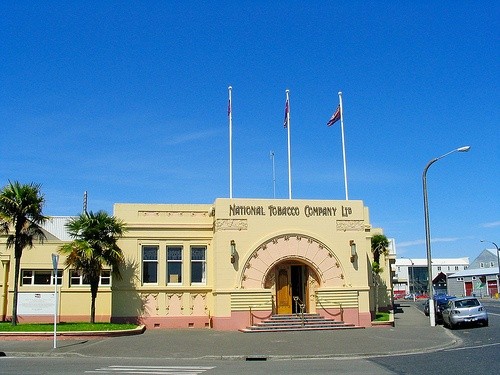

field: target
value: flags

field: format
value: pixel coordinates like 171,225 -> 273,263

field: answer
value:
227,98 -> 230,115
284,99 -> 289,127
326,104 -> 340,126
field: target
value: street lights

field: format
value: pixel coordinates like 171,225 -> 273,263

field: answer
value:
401,256 -> 415,301
481,240 -> 500,294
423,145 -> 472,327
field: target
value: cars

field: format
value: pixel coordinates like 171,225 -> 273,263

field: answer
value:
393,293 -> 418,300
423,294 -> 488,330
